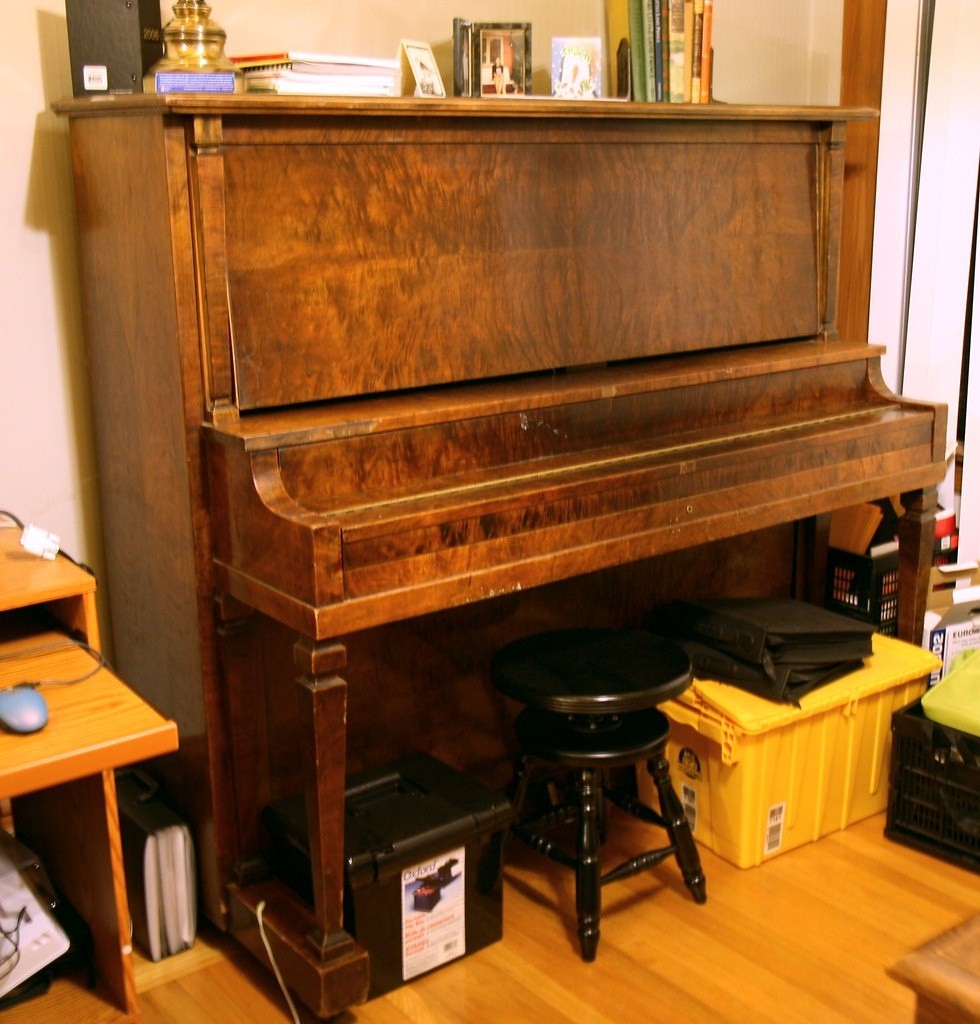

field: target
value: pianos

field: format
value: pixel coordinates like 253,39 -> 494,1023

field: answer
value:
49,85 -> 950,1021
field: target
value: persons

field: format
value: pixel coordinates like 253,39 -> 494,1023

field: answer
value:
491,57 -> 507,94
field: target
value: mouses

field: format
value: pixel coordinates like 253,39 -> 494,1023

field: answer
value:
0,687 -> 48,733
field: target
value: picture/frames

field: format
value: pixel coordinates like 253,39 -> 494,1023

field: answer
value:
473,22 -> 532,97
453,17 -> 472,97
403,41 -> 446,98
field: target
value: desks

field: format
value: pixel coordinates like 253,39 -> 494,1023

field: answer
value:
0,526 -> 179,1015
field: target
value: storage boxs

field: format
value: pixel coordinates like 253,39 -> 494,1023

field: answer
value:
921,561 -> 980,691
638,632 -> 944,870
825,547 -> 899,638
884,695 -> 980,877
258,751 -> 514,999
895,508 -> 959,592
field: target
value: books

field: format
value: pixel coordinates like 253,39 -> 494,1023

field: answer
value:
229,52 -> 403,99
65,0 -> 165,101
116,772 -> 198,964
604,0 -> 713,104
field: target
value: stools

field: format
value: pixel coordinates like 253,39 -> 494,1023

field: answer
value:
489,628 -> 706,960
